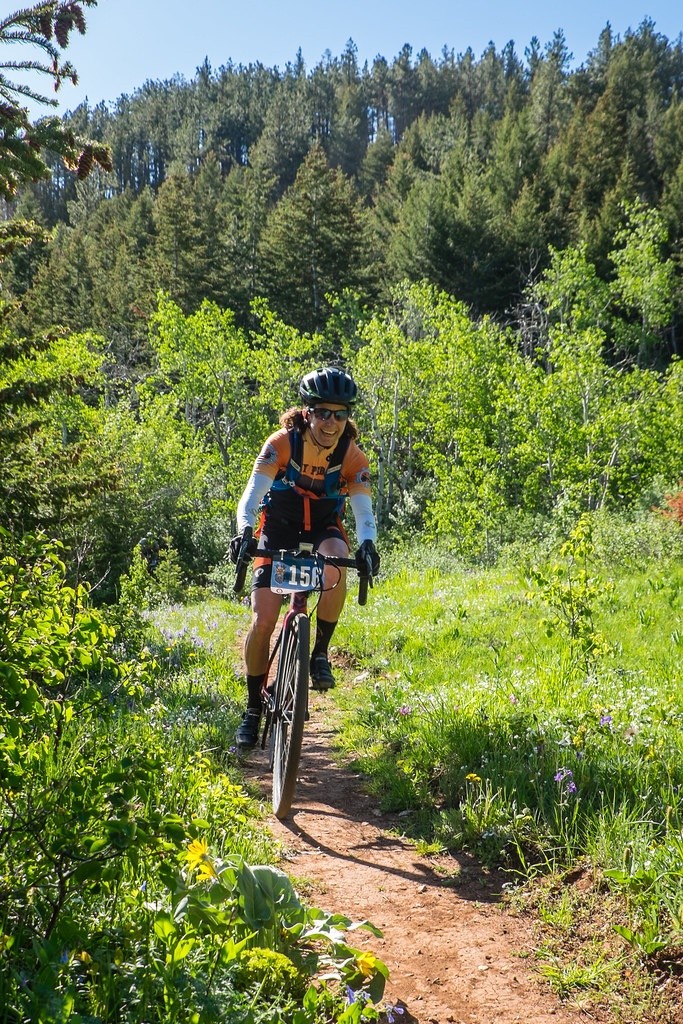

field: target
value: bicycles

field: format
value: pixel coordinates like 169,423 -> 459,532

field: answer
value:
231,527 -> 373,819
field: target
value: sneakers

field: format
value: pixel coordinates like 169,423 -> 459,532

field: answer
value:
233,707 -> 258,748
310,650 -> 335,689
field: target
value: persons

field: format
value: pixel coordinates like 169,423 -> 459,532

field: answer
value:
229,367 -> 380,750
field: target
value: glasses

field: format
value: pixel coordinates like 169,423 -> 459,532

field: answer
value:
308,407 -> 348,422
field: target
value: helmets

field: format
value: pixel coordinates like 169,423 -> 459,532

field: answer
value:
300,367 -> 358,406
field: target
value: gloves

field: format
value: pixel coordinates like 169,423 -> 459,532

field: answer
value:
229,535 -> 258,564
355,540 -> 381,579
